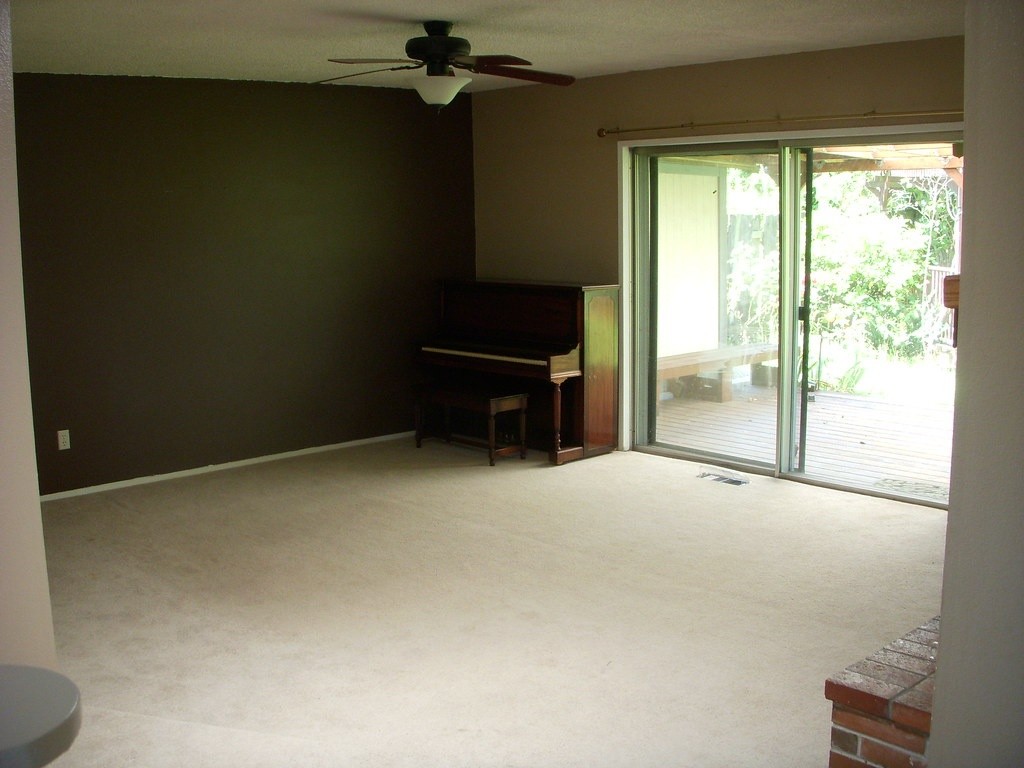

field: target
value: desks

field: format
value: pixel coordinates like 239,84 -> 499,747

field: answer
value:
0,662 -> 83,768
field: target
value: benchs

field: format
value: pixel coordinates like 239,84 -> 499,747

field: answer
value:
656,345 -> 801,409
412,380 -> 531,467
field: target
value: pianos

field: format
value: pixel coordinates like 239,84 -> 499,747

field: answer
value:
413,275 -> 623,469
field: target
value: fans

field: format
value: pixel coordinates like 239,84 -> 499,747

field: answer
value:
310,19 -> 576,86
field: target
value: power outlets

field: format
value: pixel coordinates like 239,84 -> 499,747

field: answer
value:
57,429 -> 70,450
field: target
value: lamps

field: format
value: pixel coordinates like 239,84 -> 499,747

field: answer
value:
404,62 -> 472,114
